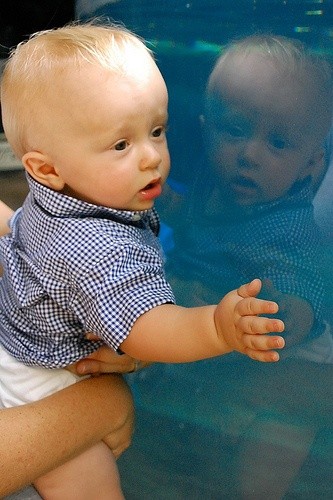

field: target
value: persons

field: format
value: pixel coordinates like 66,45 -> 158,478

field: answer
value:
0,25 -> 288,500
1,199 -> 140,500
150,34 -> 333,498
